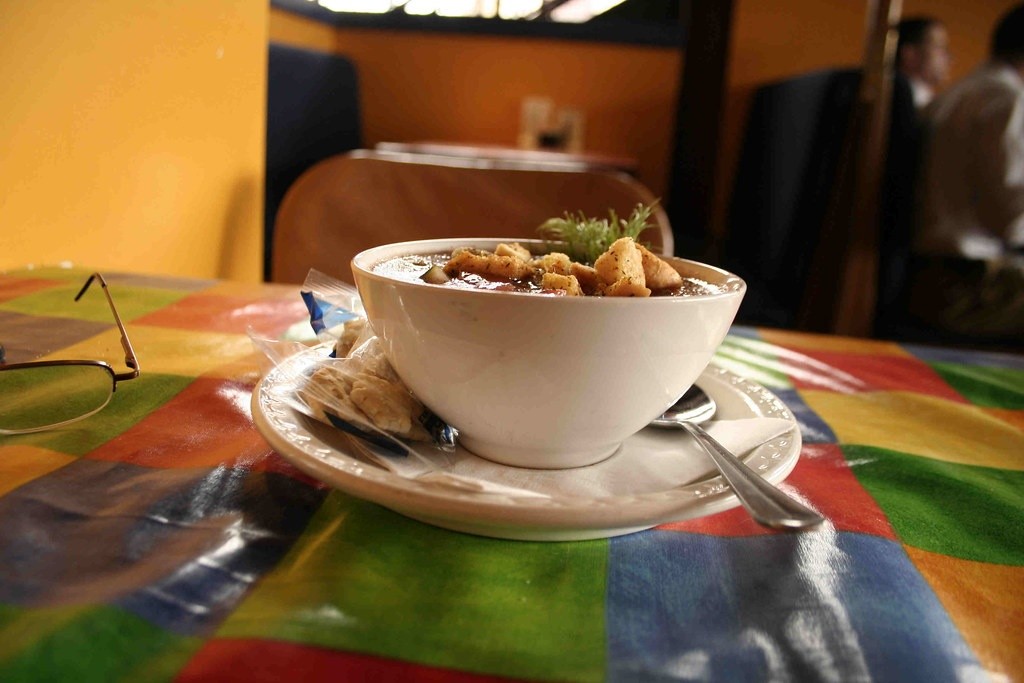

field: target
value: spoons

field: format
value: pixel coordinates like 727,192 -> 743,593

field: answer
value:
648,382 -> 824,530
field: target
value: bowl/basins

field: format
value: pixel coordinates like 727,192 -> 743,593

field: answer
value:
351,238 -> 746,470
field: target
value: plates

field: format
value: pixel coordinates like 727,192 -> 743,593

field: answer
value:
250,338 -> 806,541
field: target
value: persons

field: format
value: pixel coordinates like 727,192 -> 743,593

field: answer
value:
870,0 -> 1024,349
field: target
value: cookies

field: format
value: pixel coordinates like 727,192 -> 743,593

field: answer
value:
303,319 -> 434,439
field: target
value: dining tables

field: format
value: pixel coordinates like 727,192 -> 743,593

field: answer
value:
0,268 -> 1023,683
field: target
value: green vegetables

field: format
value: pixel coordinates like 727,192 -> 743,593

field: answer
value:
533,198 -> 661,266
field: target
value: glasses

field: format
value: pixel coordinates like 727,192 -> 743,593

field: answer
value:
0,271 -> 143,441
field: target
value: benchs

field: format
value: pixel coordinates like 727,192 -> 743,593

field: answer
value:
272,143 -> 674,284
718,65 -> 1024,361
